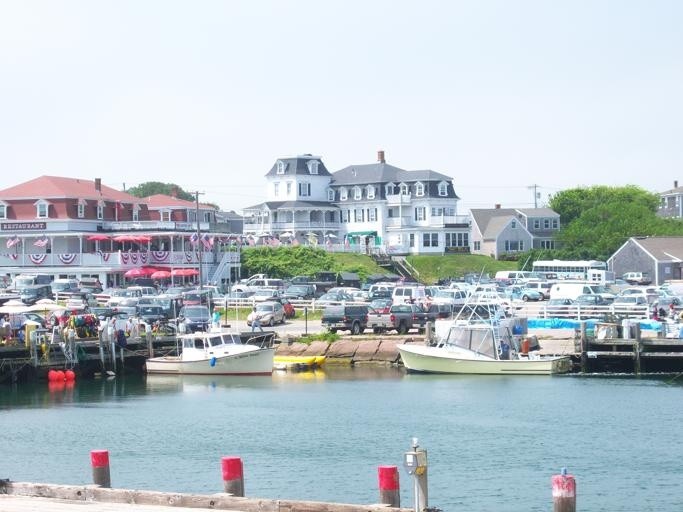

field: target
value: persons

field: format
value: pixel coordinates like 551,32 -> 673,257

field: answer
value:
212,309 -> 220,325
0,314 -> 17,341
251,306 -> 264,333
651,300 -> 683,322
110,313 -> 159,338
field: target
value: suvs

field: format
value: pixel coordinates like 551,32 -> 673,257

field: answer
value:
226,268 -> 553,319
0,273 -> 226,338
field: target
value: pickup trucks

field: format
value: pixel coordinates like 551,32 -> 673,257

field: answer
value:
367,304 -> 423,335
409,303 -> 452,335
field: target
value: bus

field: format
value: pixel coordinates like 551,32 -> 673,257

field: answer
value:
532,258 -> 606,281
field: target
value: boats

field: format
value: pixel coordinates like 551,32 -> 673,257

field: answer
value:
144,249 -> 284,375
272,353 -> 316,371
393,250 -> 572,376
291,355 -> 327,368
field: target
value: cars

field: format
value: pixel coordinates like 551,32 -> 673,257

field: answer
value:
246,301 -> 284,325
567,294 -> 611,318
608,284 -> 682,320
539,297 -> 572,319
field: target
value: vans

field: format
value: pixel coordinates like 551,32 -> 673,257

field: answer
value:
550,283 -> 614,305
621,271 -> 652,284
320,304 -> 376,335
